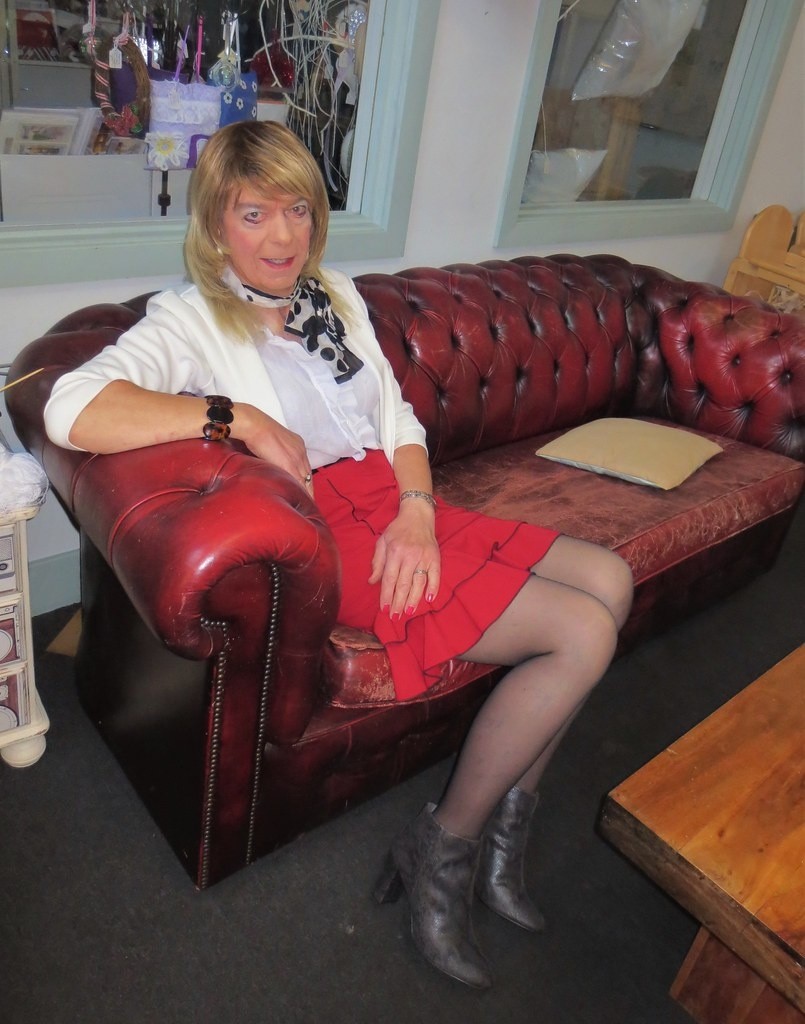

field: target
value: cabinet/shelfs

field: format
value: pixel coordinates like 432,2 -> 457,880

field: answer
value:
0,505 -> 51,767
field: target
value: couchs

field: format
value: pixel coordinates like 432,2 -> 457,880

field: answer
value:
4,254 -> 805,894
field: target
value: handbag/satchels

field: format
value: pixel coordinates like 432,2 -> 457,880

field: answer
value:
114,67 -> 189,129
256,31 -> 294,86
147,80 -> 220,173
208,71 -> 258,127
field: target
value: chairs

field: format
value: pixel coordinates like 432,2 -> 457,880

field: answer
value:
722,205 -> 805,302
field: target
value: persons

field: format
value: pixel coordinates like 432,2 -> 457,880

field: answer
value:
41,120 -> 634,989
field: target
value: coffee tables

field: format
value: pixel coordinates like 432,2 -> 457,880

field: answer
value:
599,640 -> 805,1024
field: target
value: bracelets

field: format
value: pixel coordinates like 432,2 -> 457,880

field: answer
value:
202,394 -> 233,438
399,490 -> 437,509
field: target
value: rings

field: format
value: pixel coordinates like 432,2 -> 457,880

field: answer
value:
413,570 -> 428,575
305,474 -> 311,486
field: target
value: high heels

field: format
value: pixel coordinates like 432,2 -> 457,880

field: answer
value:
477,786 -> 545,935
372,803 -> 495,990
